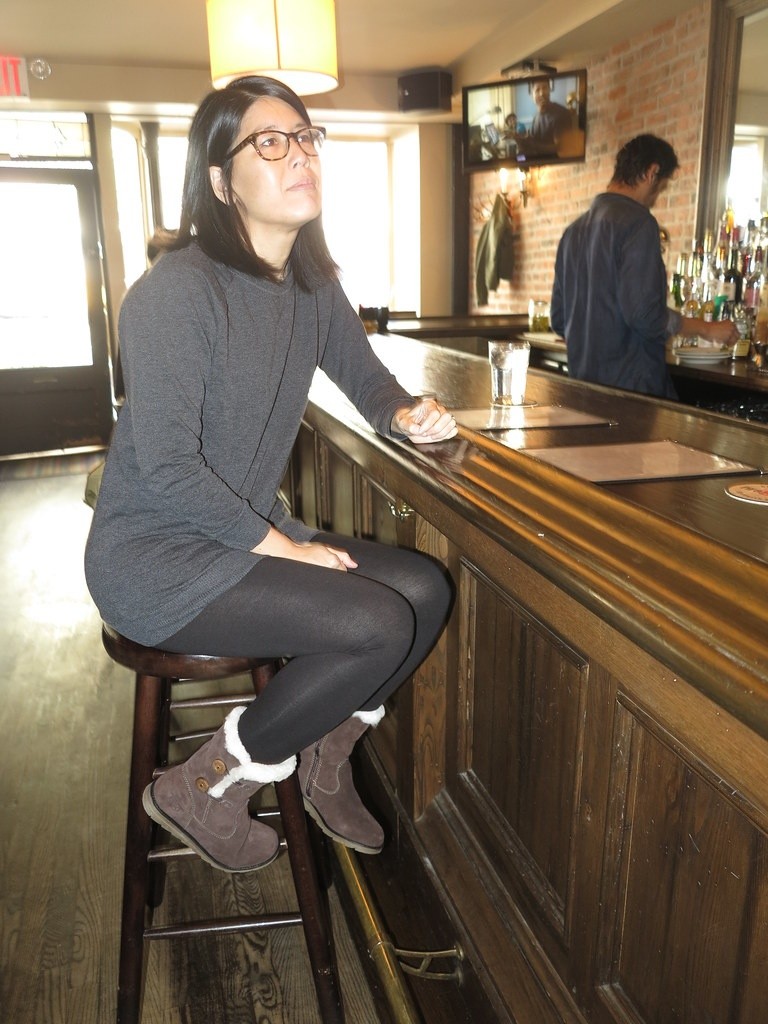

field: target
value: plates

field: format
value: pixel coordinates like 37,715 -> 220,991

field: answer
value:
673,350 -> 732,364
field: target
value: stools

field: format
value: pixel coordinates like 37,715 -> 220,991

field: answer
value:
99,623 -> 345,1024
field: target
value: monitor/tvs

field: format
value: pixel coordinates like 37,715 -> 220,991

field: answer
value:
462,68 -> 588,176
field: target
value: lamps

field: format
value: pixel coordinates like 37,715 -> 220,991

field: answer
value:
205,0 -> 340,97
498,168 -> 512,208
518,167 -> 531,206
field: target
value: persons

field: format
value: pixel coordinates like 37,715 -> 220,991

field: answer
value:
551,133 -> 742,403
510,80 -> 571,159
505,113 -> 526,137
85,75 -> 459,874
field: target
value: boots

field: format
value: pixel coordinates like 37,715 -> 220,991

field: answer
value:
142,706 -> 298,873
294,705 -> 388,854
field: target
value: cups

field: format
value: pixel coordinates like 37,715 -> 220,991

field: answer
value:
487,339 -> 531,405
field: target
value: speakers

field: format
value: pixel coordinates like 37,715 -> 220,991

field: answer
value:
397,72 -> 452,116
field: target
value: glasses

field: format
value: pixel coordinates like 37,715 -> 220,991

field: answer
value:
217,126 -> 327,168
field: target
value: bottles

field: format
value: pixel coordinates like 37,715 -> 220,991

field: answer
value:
668,203 -> 768,348
529,300 -> 549,332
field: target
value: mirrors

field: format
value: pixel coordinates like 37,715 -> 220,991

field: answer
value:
693,0 -> 768,287
